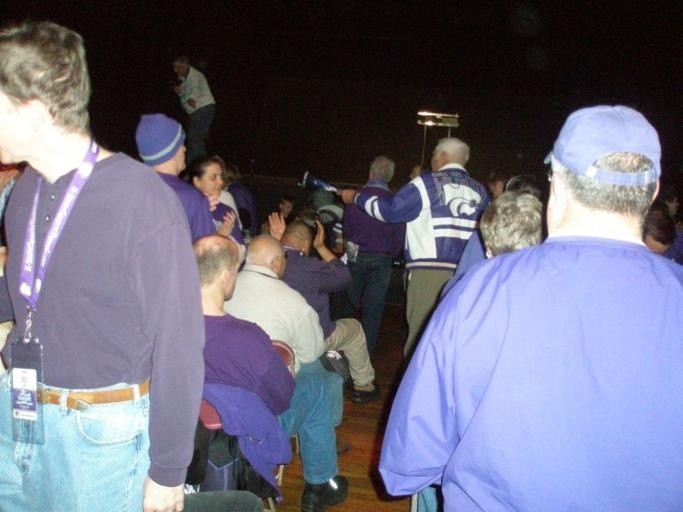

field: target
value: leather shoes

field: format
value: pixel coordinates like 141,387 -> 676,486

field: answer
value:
301,476 -> 349,511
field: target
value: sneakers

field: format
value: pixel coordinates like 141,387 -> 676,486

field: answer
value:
351,387 -> 383,405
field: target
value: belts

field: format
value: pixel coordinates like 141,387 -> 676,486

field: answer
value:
28,378 -> 149,412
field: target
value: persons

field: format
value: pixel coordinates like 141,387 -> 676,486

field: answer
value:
380,106 -> 683,512
172,54 -> 216,163
137,113 -> 683,512
2,22 -> 207,512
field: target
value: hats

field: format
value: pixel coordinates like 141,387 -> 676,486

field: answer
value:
543,103 -> 663,186
133,114 -> 185,167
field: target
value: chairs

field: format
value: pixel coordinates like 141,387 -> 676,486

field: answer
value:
271,341 -> 301,455
198,394 -> 277,512
